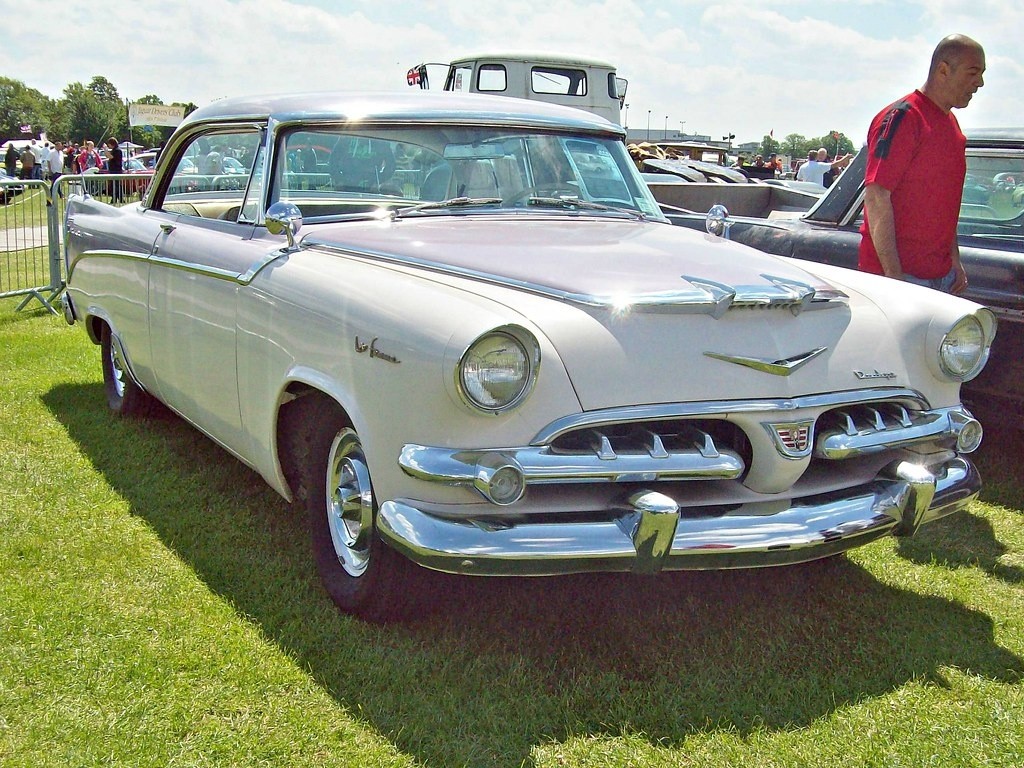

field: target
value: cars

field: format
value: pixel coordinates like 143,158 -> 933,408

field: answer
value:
73,148 -> 161,192
0,148 -> 24,179
63,89 -> 999,620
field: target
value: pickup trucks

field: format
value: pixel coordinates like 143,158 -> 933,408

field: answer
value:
644,135 -> 1023,434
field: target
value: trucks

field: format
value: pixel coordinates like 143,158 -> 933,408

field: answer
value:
407,54 -> 628,142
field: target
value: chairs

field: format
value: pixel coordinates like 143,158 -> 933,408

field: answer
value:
421,163 -> 500,212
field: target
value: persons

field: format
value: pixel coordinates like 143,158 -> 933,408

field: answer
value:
102,137 -> 127,204
795,149 -> 853,189
28,138 -> 42,189
77,140 -> 104,196
728,152 -> 784,177
198,133 -> 397,193
60,140 -> 102,178
796,147 -> 835,188
857,32 -> 987,306
4,143 -> 22,177
41,142 -> 52,183
19,145 -> 36,189
46,141 -> 66,200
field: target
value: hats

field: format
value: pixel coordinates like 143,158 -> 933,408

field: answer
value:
768,153 -> 776,159
